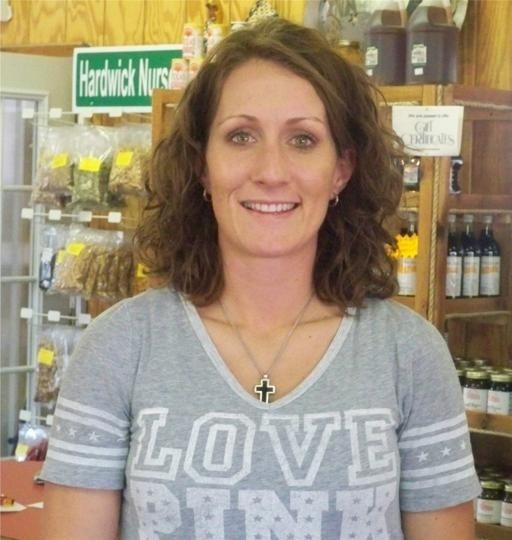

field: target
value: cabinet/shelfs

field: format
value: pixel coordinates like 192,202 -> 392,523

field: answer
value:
150,83 -> 512,540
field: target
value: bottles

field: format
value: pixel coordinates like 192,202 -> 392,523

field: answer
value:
444,213 -> 501,298
395,210 -> 417,297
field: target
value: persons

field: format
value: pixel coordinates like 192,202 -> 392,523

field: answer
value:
31,15 -> 487,540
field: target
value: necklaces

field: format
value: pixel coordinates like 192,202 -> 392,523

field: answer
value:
210,290 -> 318,410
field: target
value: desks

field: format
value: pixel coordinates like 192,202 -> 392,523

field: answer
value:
0,459 -> 46,540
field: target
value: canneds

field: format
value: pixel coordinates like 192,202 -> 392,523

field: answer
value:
454,357 -> 512,416
474,467 -> 512,527
